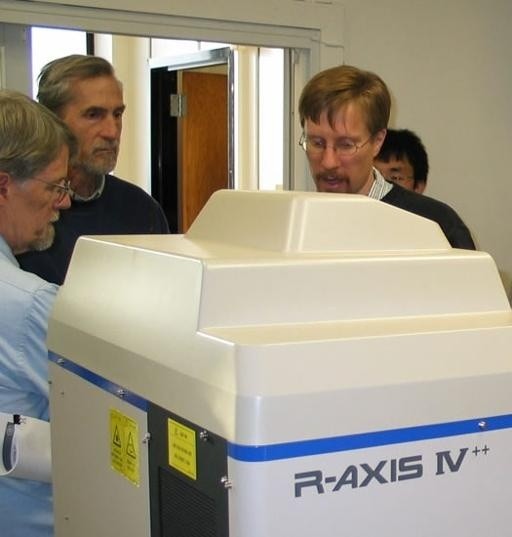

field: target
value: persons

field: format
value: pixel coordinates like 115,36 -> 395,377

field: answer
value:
298,65 -> 478,250
0,88 -> 80,536
373,124 -> 429,195
13,53 -> 173,285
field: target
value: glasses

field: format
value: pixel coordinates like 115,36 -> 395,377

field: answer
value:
298,130 -> 372,156
386,173 -> 415,186
12,172 -> 70,204
